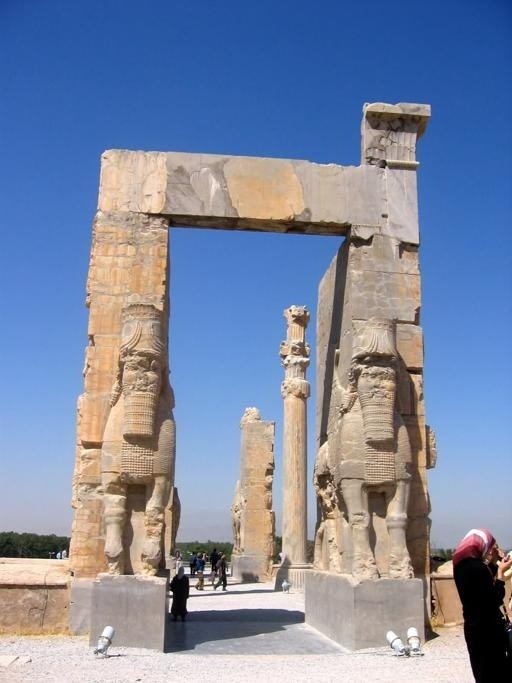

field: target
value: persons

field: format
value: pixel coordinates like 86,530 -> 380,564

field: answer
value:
56,550 -> 62,559
62,547 -> 68,559
168,546 -> 229,623
453,525 -> 512,683
